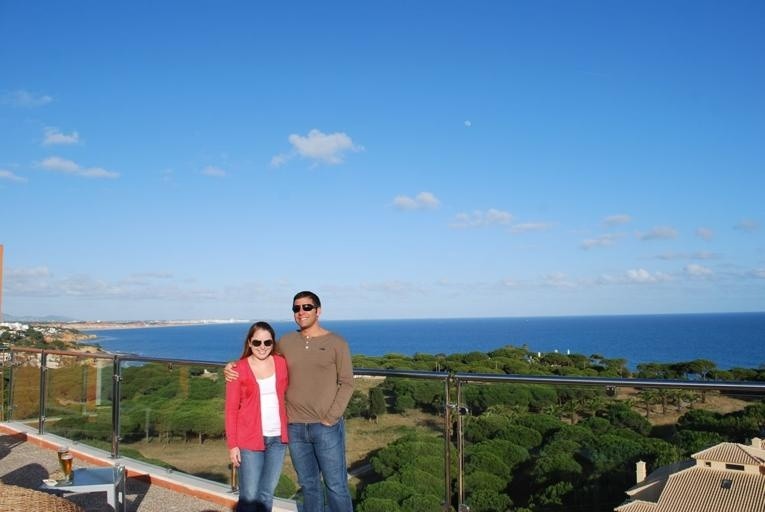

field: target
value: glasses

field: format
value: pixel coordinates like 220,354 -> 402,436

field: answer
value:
252,340 -> 273,347
293,304 -> 317,313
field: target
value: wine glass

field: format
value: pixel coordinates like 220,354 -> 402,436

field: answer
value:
57,445 -> 75,486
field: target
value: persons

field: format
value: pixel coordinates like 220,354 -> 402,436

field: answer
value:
224,291 -> 354,512
225,321 -> 288,512
